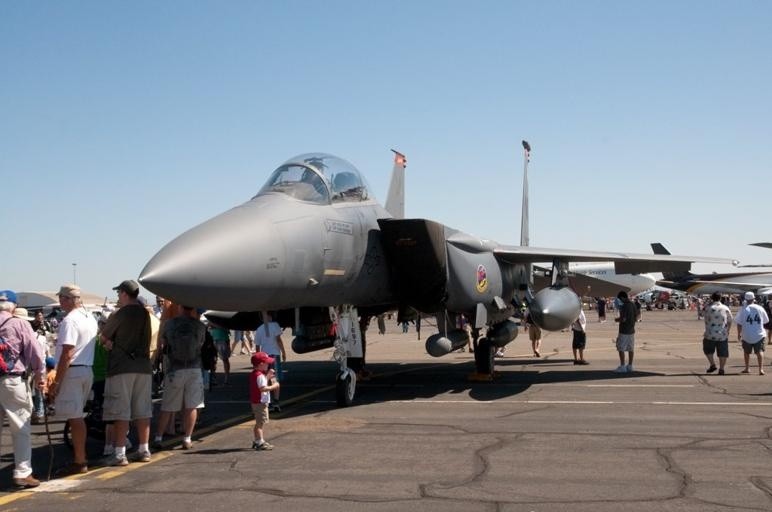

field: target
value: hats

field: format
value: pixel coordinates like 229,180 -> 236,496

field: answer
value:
0,291 -> 35,320
56,287 -> 81,298
744,293 -> 756,299
252,352 -> 275,366
113,280 -> 139,294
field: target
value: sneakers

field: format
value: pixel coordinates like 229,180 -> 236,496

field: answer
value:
253,440 -> 274,450
269,404 -> 281,413
13,439 -> 194,486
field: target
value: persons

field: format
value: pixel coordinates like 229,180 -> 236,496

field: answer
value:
154,304 -> 205,449
0,290 -> 47,486
231,330 -> 256,357
593,291 -> 772,346
100,280 -> 154,465
208,326 -> 232,384
524,308 -> 541,357
11,306 -> 63,418
572,308 -> 589,365
373,311 -> 506,357
251,352 -> 279,450
138,296 -> 200,434
46,285 -> 98,473
733,291 -> 770,375
255,310 -> 287,407
703,291 -> 733,375
92,319 -> 132,454
615,291 -> 637,373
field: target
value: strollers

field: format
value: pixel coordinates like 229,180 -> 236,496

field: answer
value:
60,371 -> 138,467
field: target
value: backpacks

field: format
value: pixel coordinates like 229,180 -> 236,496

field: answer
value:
165,318 -> 203,369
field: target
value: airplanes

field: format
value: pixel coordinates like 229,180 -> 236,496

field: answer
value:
44,141 -> 739,407
648,240 -> 772,303
532,268 -> 655,302
